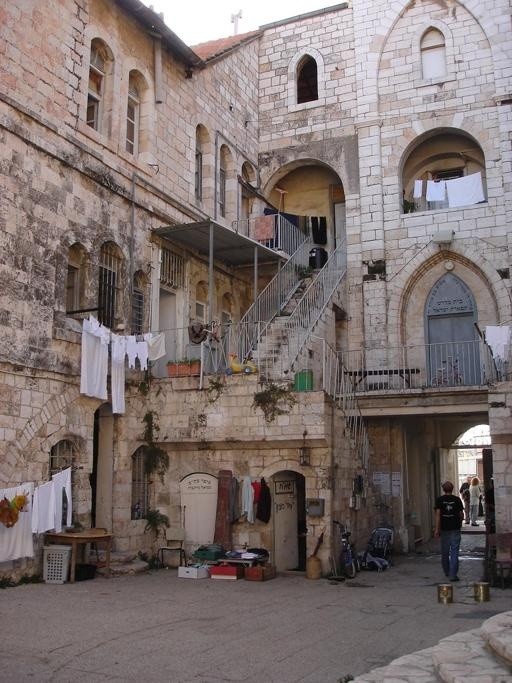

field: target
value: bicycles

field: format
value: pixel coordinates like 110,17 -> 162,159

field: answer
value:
333,520 -> 361,578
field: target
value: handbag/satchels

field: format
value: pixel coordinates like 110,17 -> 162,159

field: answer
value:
464,488 -> 470,497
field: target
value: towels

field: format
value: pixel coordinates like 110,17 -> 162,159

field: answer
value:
413,179 -> 423,199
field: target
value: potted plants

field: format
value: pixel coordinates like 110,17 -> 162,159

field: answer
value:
167,356 -> 200,377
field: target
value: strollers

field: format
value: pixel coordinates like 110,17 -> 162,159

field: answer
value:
357,526 -> 394,572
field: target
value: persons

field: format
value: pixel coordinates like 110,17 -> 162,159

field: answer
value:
460,476 -> 472,524
470,478 -> 481,526
433,481 -> 464,581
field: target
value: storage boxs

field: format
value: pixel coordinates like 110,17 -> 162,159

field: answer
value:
208,565 -> 277,581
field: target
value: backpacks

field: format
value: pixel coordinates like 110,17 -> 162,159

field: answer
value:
440,496 -> 459,530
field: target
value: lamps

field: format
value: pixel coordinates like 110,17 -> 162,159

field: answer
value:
297,431 -> 311,467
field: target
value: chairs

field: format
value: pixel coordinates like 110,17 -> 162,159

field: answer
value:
487,531 -> 512,589
155,528 -> 188,569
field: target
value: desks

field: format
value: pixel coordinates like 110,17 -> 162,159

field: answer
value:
44,526 -> 115,584
218,558 -> 256,568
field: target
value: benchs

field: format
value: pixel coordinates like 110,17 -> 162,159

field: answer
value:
344,368 -> 420,391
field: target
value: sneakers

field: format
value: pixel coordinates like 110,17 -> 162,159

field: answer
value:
448,575 -> 460,582
465,522 -> 479,527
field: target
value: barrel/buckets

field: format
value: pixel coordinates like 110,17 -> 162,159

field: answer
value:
438,583 -> 452,602
473,582 -> 489,601
295,369 -> 313,392
307,555 -> 321,579
